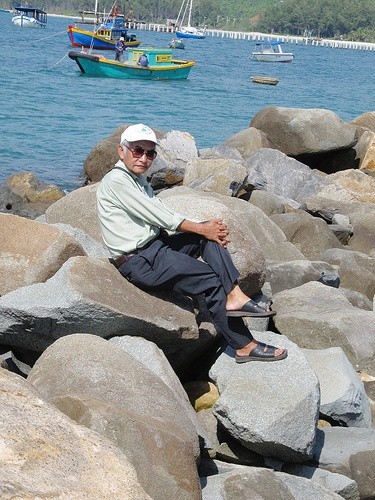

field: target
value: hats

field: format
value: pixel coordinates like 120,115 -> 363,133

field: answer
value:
120,37 -> 124,41
120,123 -> 159,145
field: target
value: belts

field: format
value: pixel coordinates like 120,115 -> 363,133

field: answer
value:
113,235 -> 162,267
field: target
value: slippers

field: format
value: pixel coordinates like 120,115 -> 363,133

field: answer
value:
235,342 -> 290,362
225,299 -> 277,318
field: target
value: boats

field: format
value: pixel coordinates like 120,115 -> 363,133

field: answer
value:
248,40 -> 295,62
175,0 -> 206,39
68,47 -> 194,81
252,76 -> 279,86
66,0 -> 141,51
169,38 -> 185,48
12,6 -> 48,29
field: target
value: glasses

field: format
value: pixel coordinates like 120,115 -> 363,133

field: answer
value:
123,145 -> 157,160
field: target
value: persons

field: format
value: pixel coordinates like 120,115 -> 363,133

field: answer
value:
115,37 -> 148,67
97,123 -> 287,364
